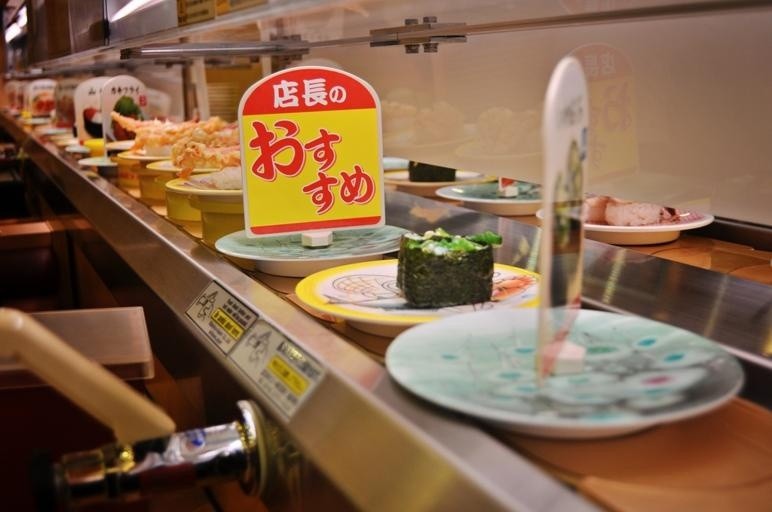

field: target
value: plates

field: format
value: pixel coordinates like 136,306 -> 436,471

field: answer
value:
294,257 -> 548,338
434,181 -> 547,218
383,170 -> 499,197
383,307 -> 748,441
548,204 -> 718,247
10,105 -> 246,216
213,222 -> 411,275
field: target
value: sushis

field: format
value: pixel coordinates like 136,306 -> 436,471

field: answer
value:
396,228 -> 503,308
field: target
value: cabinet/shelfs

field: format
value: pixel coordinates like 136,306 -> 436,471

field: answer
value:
2,79 -> 769,512
